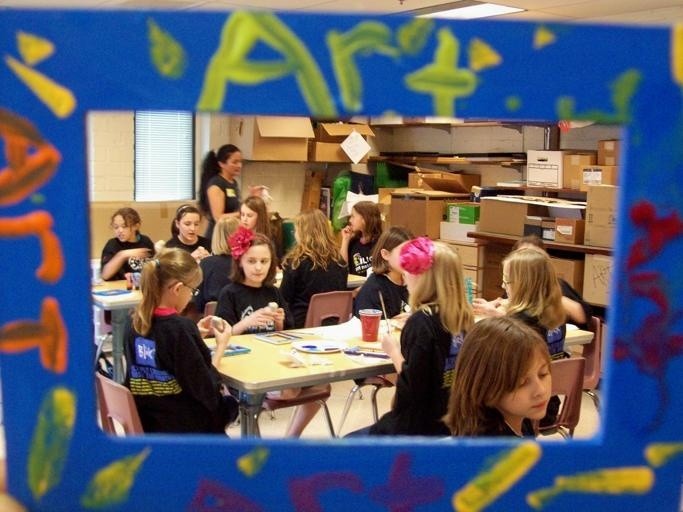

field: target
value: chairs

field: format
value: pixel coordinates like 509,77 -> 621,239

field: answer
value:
93,371 -> 146,438
529,356 -> 587,439
202,301 -> 338,440
339,286 -> 396,434
304,289 -> 354,329
583,315 -> 602,414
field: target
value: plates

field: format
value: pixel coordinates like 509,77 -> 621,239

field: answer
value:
291,340 -> 346,354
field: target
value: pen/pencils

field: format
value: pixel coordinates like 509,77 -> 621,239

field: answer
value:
377,289 -> 391,333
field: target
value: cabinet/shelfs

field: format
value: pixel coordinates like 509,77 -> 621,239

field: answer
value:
466,183 -> 615,258
436,238 -> 512,301
377,122 -> 562,199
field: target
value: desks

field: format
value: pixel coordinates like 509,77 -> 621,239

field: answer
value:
92,270 -> 368,382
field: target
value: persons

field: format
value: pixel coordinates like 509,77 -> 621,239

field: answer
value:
437,315 -> 553,435
340,200 -> 386,279
512,233 -> 593,332
340,234 -> 476,436
501,245 -> 565,436
208,225 -> 331,438
350,222 -> 416,388
166,145 -> 275,314
102,206 -> 155,325
122,246 -> 238,433
277,207 -> 349,332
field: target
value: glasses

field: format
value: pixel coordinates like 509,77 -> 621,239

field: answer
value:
168,281 -> 200,296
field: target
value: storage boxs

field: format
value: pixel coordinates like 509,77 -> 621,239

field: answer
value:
522,214 -> 553,240
476,197 -> 550,238
582,183 -> 619,248
308,140 -> 370,164
579,165 -> 619,193
550,256 -> 582,294
526,147 -> 566,189
388,188 -> 472,240
228,114 -> 317,163
581,253 -> 613,309
445,200 -> 481,225
541,221 -> 555,242
553,217 -> 585,245
596,137 -> 619,164
316,123 -> 376,143
564,149 -> 596,191
439,220 -> 477,243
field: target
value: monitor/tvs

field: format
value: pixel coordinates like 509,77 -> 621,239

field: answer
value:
350,170 -> 375,196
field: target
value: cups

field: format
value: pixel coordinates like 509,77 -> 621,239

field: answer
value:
359,309 -> 383,342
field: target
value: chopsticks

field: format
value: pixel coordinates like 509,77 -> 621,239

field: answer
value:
343,344 -> 392,363
